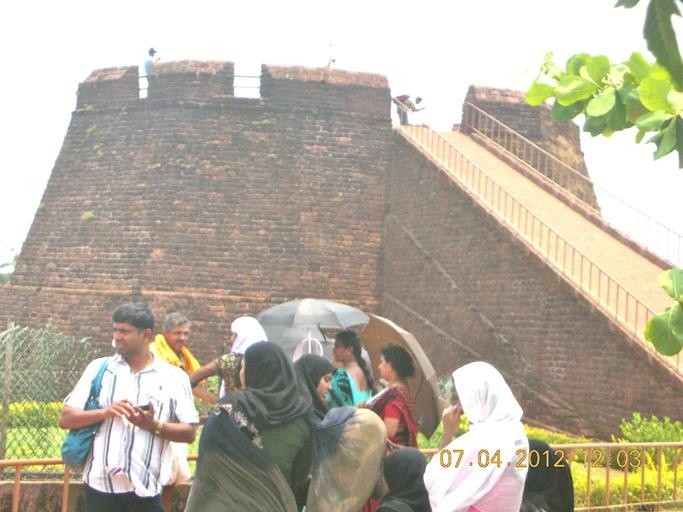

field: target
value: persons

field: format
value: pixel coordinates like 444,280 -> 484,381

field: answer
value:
59,303 -> 200,512
149,311 -> 219,512
145,48 -> 161,75
397,97 -> 425,126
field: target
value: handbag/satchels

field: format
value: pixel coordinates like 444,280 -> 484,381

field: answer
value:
61,401 -> 104,467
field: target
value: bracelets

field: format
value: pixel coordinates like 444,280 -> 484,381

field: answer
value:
151,420 -> 163,436
